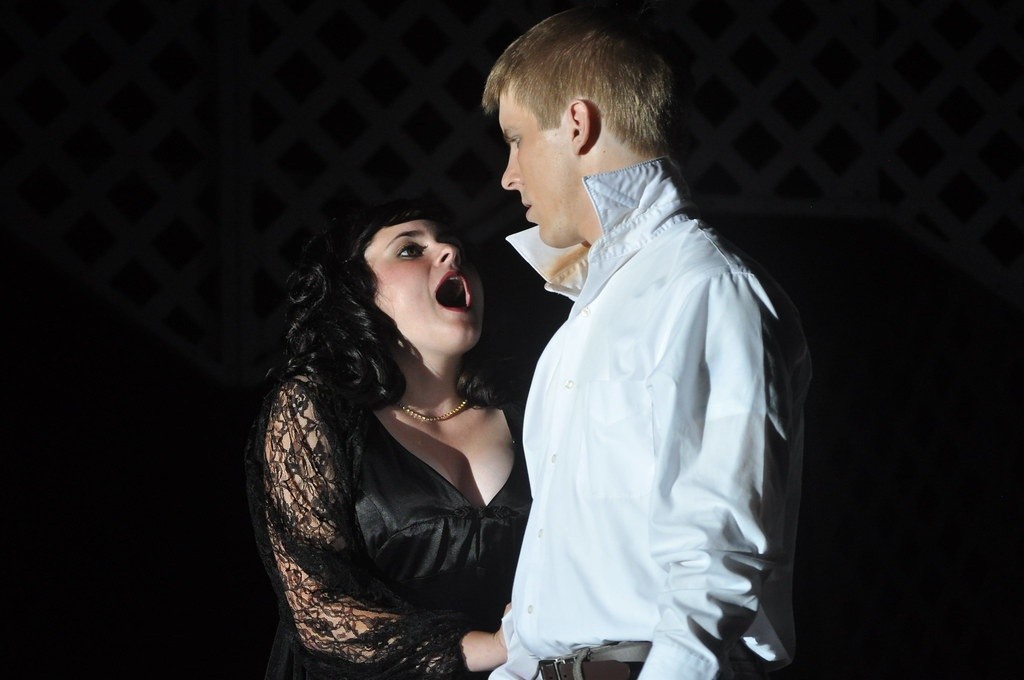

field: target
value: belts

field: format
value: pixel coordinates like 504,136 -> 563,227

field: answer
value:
539,641 -> 653,680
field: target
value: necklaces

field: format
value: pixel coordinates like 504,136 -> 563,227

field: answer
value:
378,374 -> 474,423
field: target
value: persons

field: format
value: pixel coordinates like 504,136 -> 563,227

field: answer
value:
478,2 -> 814,680
245,196 -> 533,680
